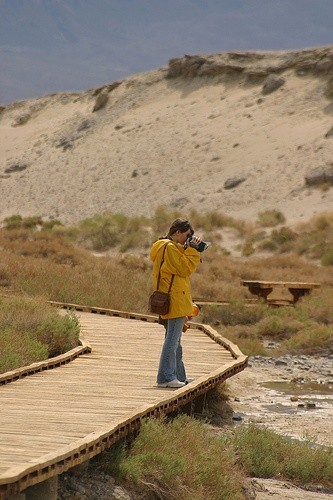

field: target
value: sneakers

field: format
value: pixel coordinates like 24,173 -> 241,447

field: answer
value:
157,378 -> 185,388
185,377 -> 195,383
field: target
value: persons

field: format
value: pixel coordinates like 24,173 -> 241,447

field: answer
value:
146,217 -> 204,388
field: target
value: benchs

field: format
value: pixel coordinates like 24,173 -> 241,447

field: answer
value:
240,279 -> 321,307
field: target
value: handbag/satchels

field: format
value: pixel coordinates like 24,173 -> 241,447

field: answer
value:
148,291 -> 171,315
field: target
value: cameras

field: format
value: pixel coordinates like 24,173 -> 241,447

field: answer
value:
184,235 -> 208,253
